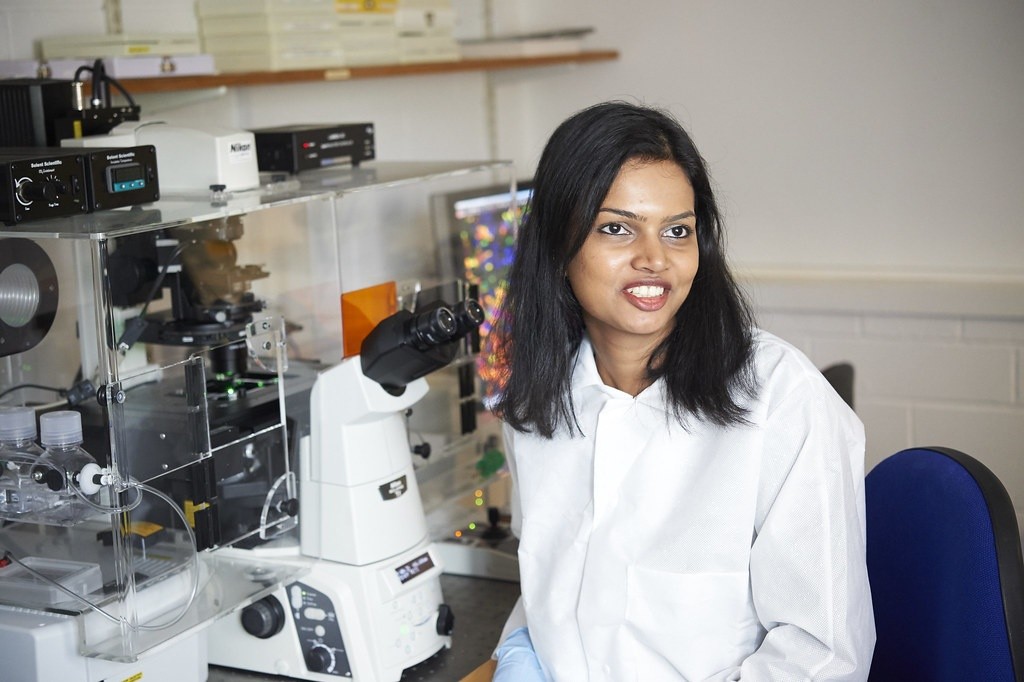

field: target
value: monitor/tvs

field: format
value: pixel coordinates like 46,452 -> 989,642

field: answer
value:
432,177 -> 536,412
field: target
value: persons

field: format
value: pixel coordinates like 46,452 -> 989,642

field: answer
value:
491,103 -> 876,682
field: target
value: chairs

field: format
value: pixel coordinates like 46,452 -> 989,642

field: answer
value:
865,446 -> 1024,682
